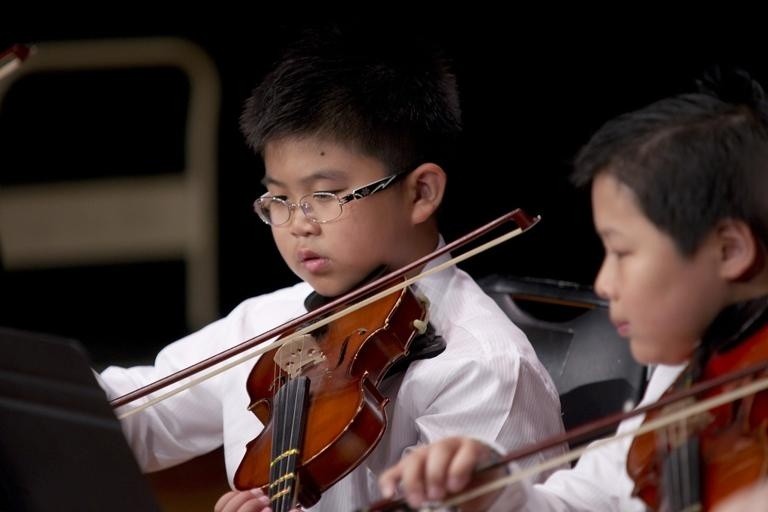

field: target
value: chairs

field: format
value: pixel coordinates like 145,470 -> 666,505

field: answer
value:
473,269 -> 654,463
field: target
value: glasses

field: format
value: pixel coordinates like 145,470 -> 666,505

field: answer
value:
252,170 -> 409,228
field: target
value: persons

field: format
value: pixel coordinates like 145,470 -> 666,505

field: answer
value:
80,30 -> 570,512
378,92 -> 768,511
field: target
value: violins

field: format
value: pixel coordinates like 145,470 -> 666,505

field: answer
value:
627,298 -> 768,512
231,269 -> 446,510
